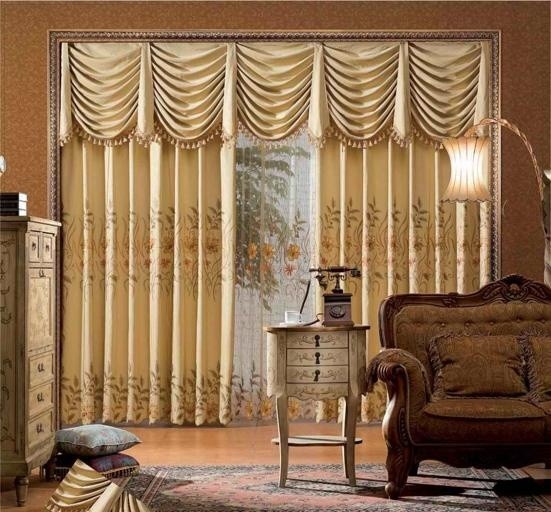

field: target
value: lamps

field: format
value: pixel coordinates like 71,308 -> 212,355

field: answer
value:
439,117 -> 544,205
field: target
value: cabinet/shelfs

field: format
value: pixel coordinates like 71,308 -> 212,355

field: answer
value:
0,214 -> 62,506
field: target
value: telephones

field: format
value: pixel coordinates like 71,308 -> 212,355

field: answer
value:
308,267 -> 362,325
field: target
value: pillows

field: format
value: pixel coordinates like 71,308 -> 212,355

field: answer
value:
56,424 -> 141,457
519,328 -> 551,402
41,452 -> 141,480
428,332 -> 530,403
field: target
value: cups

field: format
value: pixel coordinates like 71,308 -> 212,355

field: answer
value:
284,311 -> 303,324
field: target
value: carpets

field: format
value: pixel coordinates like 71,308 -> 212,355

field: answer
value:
106,464 -> 551,512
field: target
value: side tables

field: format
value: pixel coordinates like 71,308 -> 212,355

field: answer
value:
264,325 -> 373,488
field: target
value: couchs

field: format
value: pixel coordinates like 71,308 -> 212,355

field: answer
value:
377,272 -> 551,498
